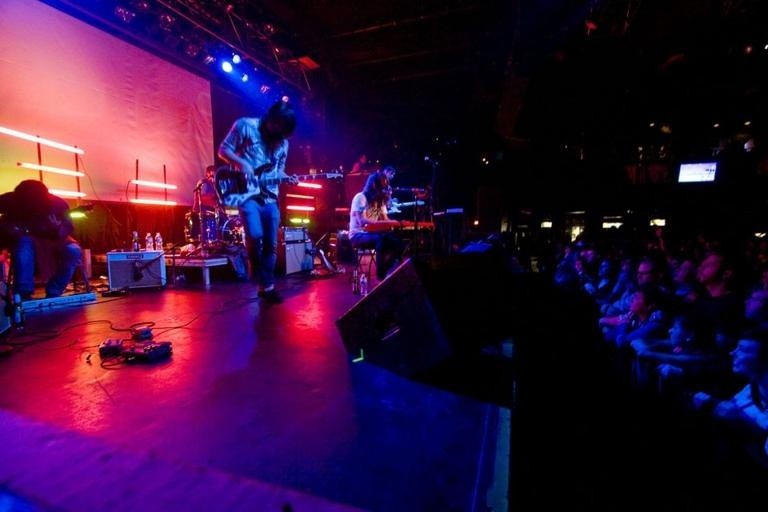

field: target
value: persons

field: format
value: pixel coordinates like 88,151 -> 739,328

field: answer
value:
219,99 -> 298,304
552,233 -> 766,434
364,164 -> 395,212
348,172 -> 401,278
1,180 -> 82,299
194,164 -> 229,221
350,153 -> 368,174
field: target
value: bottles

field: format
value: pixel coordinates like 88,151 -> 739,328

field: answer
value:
201,240 -> 209,258
121,232 -> 163,252
352,269 -> 358,295
12,293 -> 24,330
359,273 -> 368,297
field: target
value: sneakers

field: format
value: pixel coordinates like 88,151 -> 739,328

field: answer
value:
265,289 -> 283,305
257,285 -> 265,297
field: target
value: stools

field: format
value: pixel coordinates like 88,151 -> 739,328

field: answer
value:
352,248 -> 377,274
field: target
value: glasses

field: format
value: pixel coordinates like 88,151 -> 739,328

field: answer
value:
638,271 -> 654,275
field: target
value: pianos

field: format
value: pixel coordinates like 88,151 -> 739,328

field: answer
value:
363,220 -> 433,232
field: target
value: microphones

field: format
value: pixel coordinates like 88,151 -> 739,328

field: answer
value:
422,154 -> 440,169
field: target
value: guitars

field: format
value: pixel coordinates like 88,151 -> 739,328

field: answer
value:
215,166 -> 344,207
386,198 -> 425,214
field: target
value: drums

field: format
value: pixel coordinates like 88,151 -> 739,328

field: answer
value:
184,211 -> 216,243
222,217 -> 247,247
